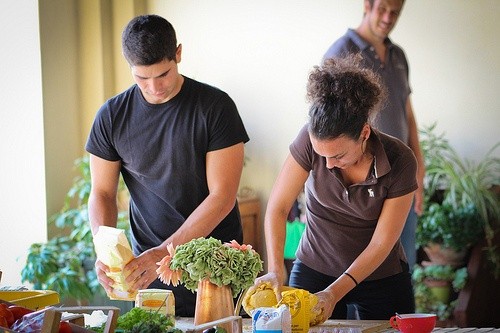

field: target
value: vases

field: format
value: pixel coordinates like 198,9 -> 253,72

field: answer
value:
191,280 -> 236,333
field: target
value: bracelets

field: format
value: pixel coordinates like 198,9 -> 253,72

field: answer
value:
343,272 -> 358,286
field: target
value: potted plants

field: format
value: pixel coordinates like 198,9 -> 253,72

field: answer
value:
413,266 -> 467,302
409,201 -> 484,314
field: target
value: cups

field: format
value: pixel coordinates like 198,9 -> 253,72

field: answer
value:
390,313 -> 437,333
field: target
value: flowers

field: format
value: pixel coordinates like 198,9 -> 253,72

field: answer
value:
157,236 -> 264,290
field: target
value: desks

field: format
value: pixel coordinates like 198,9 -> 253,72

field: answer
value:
174,317 -> 500,333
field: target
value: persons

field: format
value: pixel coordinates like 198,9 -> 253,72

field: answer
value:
322,0 -> 423,288
85,13 -> 254,319
241,51 -> 417,327
